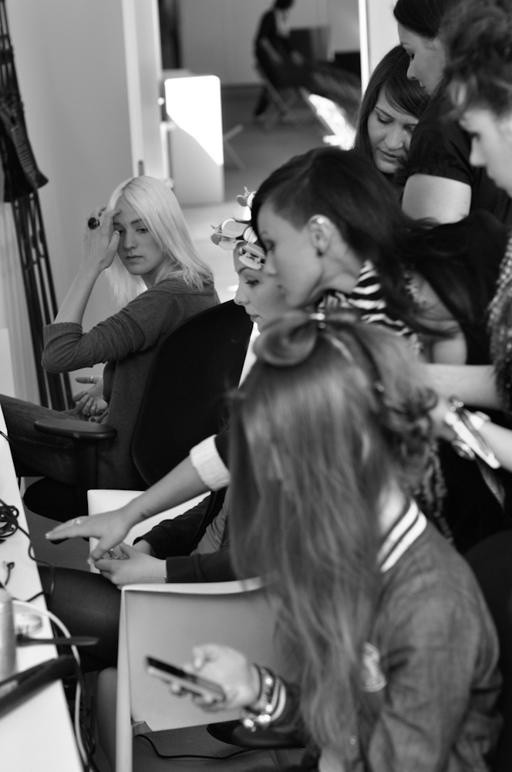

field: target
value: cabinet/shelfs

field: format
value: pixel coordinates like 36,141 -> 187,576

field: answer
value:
162,67 -> 225,206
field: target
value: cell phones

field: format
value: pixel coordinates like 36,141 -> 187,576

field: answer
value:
141,654 -> 229,705
443,391 -> 503,474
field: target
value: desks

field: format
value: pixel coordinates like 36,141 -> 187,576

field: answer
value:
0,396 -> 78,772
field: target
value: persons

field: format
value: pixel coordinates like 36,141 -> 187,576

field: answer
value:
356,1 -> 512,771
41,149 -> 438,770
164,315 -> 502,772
0,174 -> 219,528
255,1 -> 302,120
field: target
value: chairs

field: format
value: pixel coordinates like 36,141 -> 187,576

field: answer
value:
251,49 -> 362,135
22,298 -> 512,772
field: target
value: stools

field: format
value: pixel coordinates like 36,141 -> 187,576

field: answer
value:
223,122 -> 247,171
